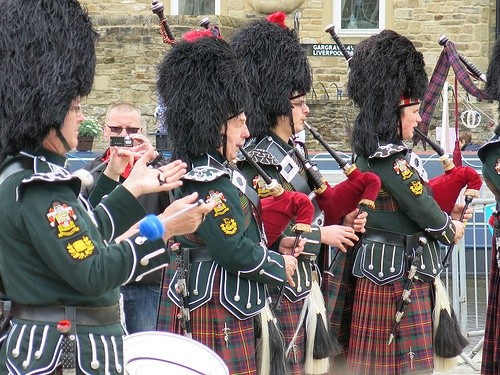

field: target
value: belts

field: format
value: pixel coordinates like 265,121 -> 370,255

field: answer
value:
172,246 -> 213,263
7,300 -> 121,326
361,224 -> 427,248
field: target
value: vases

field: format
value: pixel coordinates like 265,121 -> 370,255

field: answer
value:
76,136 -> 94,151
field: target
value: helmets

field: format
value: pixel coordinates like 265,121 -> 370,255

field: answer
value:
346,29 -> 429,160
230,11 -> 314,138
156,26 -> 250,173
0,0 -> 96,166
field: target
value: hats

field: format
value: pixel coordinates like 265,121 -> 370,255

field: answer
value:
484,38 -> 500,128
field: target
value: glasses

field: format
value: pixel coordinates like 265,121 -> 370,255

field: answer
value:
106,123 -> 141,134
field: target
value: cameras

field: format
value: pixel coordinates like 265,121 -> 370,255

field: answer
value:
110,136 -> 133,147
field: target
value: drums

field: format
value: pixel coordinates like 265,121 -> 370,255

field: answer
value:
121,330 -> 230,375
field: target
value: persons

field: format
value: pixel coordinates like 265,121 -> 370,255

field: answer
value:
82,104 -> 169,335
325,73 -> 473,374
153,36 -> 303,375
229,74 -> 368,374
476,98 -> 500,375
0,72 -> 214,375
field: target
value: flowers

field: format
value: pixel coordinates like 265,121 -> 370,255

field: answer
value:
78,115 -> 103,136
